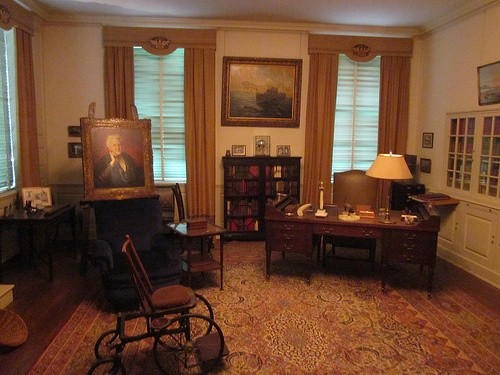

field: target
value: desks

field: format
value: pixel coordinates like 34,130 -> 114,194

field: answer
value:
0,203 -> 77,283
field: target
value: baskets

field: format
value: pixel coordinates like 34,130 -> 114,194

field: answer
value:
0,309 -> 27,347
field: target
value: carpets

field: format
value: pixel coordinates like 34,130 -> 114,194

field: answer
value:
26,238 -> 500,375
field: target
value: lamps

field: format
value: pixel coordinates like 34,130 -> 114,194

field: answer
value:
365,150 -> 413,225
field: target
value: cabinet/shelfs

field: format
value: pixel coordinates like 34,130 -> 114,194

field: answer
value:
222,156 -> 302,241
262,202 -> 440,295
167,218 -> 226,291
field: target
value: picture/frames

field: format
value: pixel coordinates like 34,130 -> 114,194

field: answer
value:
232,145 -> 246,156
420,158 -> 431,175
220,55 -> 303,129
422,132 -> 434,148
254,135 -> 271,156
79,116 -> 160,202
20,186 -> 52,208
277,144 -> 291,157
477,60 -> 500,106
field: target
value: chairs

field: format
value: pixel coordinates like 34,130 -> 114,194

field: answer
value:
322,170 -> 382,275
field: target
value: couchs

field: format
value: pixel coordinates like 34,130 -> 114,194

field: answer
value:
88,196 -> 182,313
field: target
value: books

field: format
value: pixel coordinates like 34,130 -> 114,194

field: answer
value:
359,211 -> 374,218
418,192 -> 449,201
227,200 -> 258,216
228,180 -> 297,194
227,218 -> 258,231
228,165 -> 295,178
